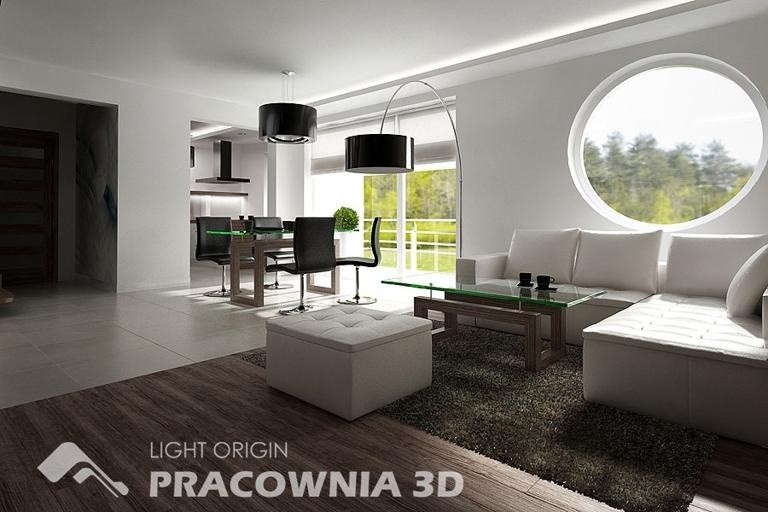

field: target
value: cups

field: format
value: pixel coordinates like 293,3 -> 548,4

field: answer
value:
519,288 -> 532,300
536,275 -> 555,288
519,272 -> 532,284
537,293 -> 555,304
241,220 -> 253,231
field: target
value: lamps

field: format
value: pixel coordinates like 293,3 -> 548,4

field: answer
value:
345,81 -> 463,258
259,70 -> 317,144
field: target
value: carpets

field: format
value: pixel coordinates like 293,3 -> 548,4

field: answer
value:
242,317 -> 721,512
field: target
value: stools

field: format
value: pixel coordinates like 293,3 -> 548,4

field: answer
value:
266,305 -> 433,421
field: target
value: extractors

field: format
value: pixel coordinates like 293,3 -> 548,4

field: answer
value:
194,140 -> 251,185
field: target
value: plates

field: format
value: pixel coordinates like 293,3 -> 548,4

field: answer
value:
535,287 -> 558,292
516,283 -> 535,287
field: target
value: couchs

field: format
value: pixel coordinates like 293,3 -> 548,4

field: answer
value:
457,252 -> 767,448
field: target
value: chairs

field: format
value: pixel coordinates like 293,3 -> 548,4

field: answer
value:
335,217 -> 381,305
266,217 -> 335,315
196,217 -> 231,297
283,221 -> 296,231
253,217 -> 294,289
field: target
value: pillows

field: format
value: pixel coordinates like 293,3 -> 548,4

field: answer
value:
572,231 -> 662,294
503,228 -> 580,284
666,234 -> 768,297
726,244 -> 767,316
